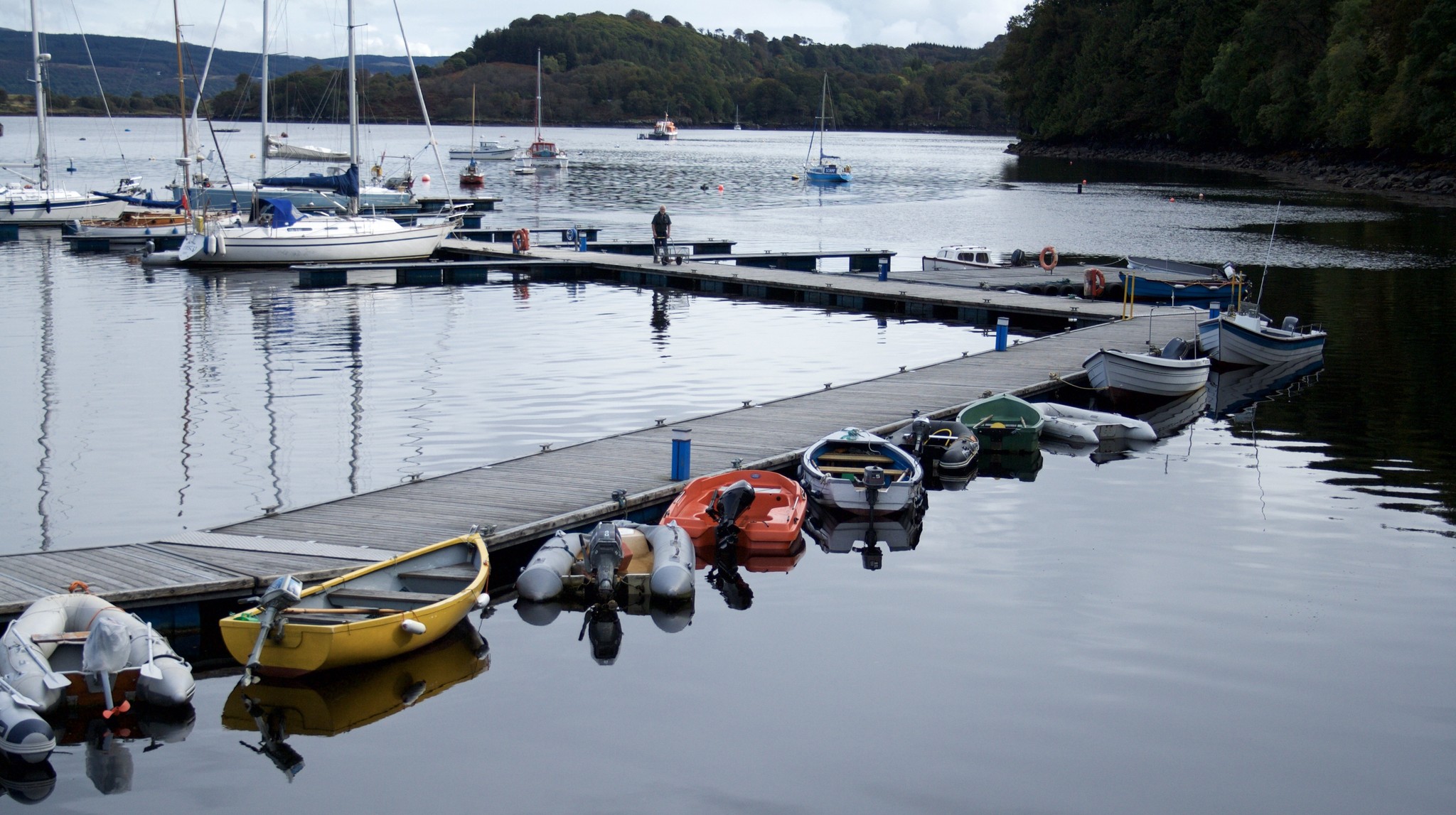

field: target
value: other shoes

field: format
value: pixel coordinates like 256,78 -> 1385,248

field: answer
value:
667,260 -> 673,263
654,260 -> 660,264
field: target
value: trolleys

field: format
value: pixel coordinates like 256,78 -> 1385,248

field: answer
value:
651,236 -> 690,266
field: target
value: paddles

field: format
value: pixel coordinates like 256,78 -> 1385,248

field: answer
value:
281,608 -> 404,615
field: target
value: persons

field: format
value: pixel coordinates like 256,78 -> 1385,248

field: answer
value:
651,205 -> 672,263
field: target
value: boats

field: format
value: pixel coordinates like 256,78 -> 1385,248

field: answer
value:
1082,305 -> 1212,406
801,497 -> 928,571
1088,355 -> 1324,439
1119,200 -> 1327,368
922,245 -> 1037,271
222,615 -> 490,783
886,393 -> 1157,477
0,592 -> 196,766
648,105 -> 678,140
659,469 -> 807,557
449,141 -> 537,175
516,518 -> 694,615
219,524 -> 493,687
798,425 -> 929,518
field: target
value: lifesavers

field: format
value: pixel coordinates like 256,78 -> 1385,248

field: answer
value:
1039,245 -> 1058,271
1087,268 -> 1105,296
559,151 -> 565,156
513,229 -> 526,251
371,166 -> 382,176
526,150 -> 532,156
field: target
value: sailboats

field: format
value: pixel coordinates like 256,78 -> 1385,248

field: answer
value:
803,70 -> 853,182
734,104 -> 741,130
0,0 -> 140,224
514,47 -> 569,168
60,0 -> 485,267
